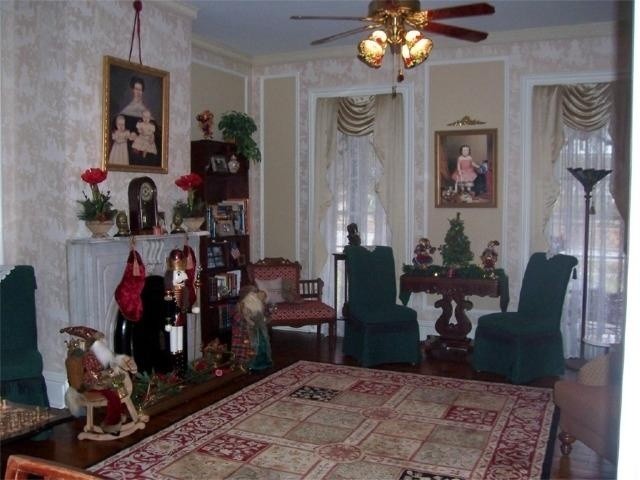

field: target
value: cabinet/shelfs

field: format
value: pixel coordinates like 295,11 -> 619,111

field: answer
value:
194,145 -> 249,356
63,233 -> 215,404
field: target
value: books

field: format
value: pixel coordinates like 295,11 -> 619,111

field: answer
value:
207,198 -> 248,329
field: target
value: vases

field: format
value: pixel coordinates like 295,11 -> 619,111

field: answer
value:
181,216 -> 205,232
85,215 -> 114,237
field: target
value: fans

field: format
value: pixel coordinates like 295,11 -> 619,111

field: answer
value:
287,0 -> 495,47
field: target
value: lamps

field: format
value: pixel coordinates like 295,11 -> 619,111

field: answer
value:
358,3 -> 432,98
566,164 -> 610,364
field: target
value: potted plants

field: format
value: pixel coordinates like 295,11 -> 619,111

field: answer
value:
217,107 -> 263,163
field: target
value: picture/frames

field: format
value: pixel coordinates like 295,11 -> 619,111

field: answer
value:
98,52 -> 170,174
434,116 -> 498,209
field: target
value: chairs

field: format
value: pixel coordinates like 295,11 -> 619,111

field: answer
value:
553,344 -> 623,468
247,255 -> 337,356
3,454 -> 106,480
343,244 -> 422,369
0,264 -> 55,442
470,250 -> 578,378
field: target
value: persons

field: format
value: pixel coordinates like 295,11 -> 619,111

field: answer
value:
59,326 -> 150,441
132,111 -> 157,159
111,76 -> 160,165
164,249 -> 189,313
452,146 -> 478,194
109,116 -> 131,164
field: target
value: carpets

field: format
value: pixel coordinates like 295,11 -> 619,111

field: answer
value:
81,356 -> 558,478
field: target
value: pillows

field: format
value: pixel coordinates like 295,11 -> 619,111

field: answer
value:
255,275 -> 296,305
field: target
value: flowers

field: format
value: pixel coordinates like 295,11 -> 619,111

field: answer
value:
196,108 -> 214,139
172,175 -> 207,218
136,364 -> 185,398
187,338 -> 236,379
78,164 -> 115,220
